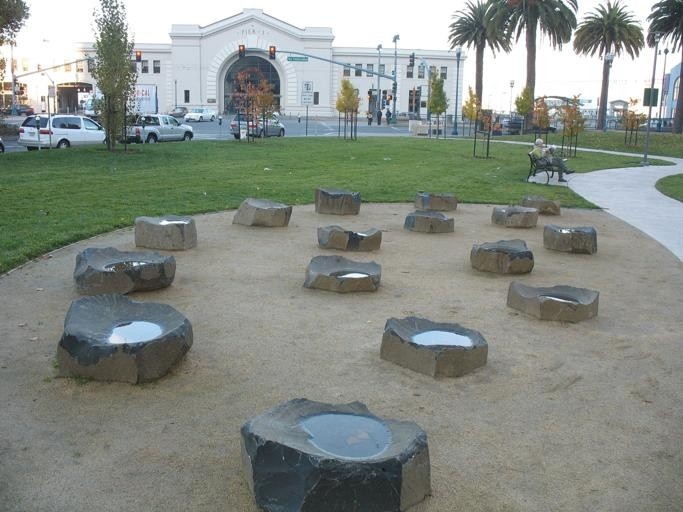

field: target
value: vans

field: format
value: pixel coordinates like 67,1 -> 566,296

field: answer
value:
18,114 -> 107,150
119,113 -> 194,143
83,93 -> 104,118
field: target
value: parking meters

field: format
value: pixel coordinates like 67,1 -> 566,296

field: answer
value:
36,116 -> 41,150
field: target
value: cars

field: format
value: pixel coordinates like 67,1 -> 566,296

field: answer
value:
502,118 -> 557,135
169,107 -> 188,118
2,104 -> 35,116
229,112 -> 285,139
184,108 -> 217,122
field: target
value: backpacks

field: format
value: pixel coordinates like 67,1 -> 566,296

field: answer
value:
536,158 -> 549,166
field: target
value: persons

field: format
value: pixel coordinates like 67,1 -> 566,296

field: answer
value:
366,112 -> 372,126
532,139 -> 575,182
296,112 -> 301,124
384,108 -> 392,126
376,110 -> 382,126
656,122 -> 661,132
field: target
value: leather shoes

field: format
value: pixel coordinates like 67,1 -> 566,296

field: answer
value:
566,169 -> 575,174
558,178 -> 566,183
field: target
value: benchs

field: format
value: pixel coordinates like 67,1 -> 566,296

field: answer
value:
528,151 -> 554,184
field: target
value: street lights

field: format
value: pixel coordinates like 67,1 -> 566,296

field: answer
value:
642,29 -> 662,166
452,47 -> 462,136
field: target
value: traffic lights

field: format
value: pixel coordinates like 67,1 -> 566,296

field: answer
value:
387,94 -> 393,101
238,44 -> 246,59
135,50 -> 142,62
367,91 -> 373,99
269,46 -> 276,60
409,52 -> 415,66
